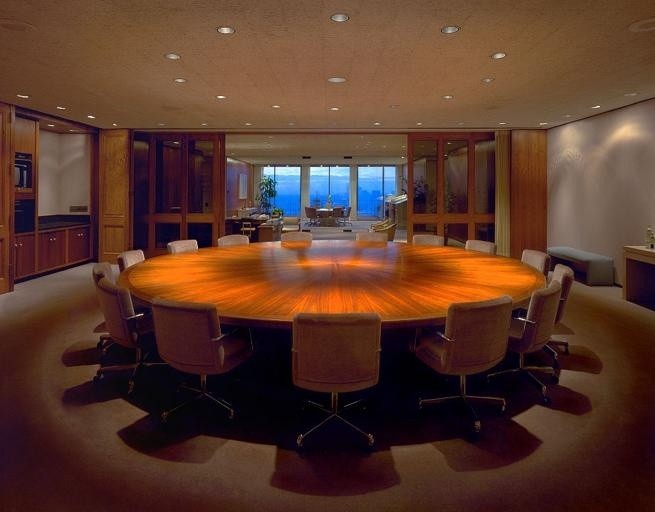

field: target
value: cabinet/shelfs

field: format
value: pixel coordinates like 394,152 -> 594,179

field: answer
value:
37,228 -> 67,274
67,224 -> 92,267
15,112 -> 41,281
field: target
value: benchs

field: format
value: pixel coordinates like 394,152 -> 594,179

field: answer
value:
547,245 -> 615,288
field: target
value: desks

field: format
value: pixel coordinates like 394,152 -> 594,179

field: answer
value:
115,240 -> 549,427
623,245 -> 654,306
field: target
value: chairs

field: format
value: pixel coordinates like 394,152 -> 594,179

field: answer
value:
412,234 -> 444,247
216,235 -> 249,246
92,261 -> 148,359
225,200 -> 410,241
146,298 -> 254,419
465,240 -> 497,255
167,239 -> 198,255
485,280 -> 563,402
407,293 -> 515,434
509,263 -> 574,364
93,279 -> 166,393
522,249 -> 550,275
118,249 -> 146,270
290,312 -> 382,450
